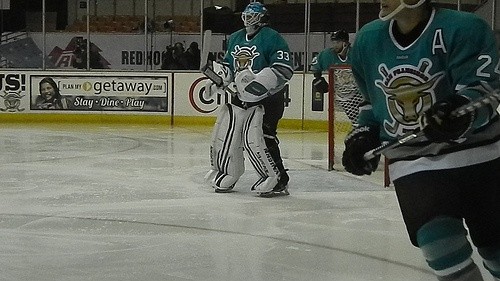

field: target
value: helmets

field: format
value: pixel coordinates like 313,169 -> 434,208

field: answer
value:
241,2 -> 270,27
331,29 -> 349,42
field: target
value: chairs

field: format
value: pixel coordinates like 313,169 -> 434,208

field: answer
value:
65,15 -> 201,33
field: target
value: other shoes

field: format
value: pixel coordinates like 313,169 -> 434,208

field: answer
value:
273,172 -> 289,191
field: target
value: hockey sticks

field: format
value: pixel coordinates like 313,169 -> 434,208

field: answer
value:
200,65 -> 273,129
363,78 -> 500,163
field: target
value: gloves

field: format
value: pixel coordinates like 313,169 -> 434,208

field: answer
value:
313,77 -> 329,93
342,124 -> 382,176
423,95 -> 472,143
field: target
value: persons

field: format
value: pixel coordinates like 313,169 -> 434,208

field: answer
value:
36,79 -> 68,109
310,28 -> 352,71
342,0 -> 500,281
162,42 -> 199,71
72,38 -> 102,69
204,2 -> 294,196
